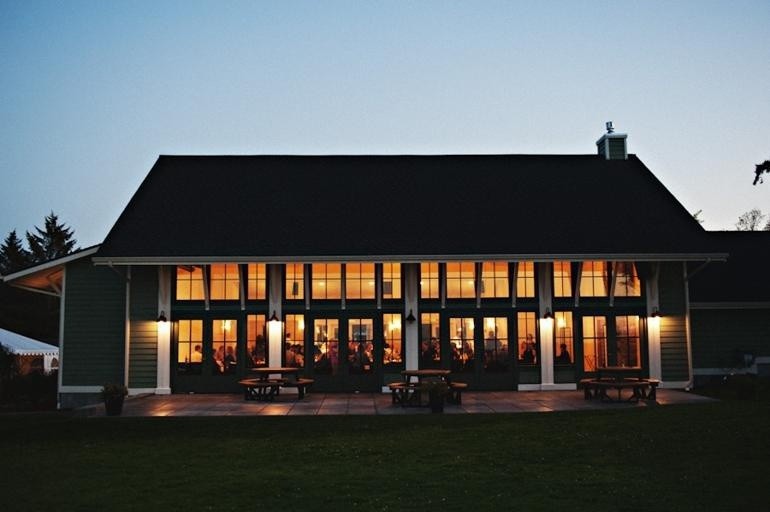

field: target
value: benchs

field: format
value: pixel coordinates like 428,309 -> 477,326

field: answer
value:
576,377 -> 659,402
389,380 -> 468,408
239,377 -> 314,403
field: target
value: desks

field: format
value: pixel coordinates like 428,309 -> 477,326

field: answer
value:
250,367 -> 299,397
597,367 -> 642,399
401,369 -> 451,406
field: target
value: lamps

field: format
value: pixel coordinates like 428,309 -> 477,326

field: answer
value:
406,308 -> 416,324
156,310 -> 168,323
651,307 -> 664,317
269,310 -> 280,322
543,307 -> 555,320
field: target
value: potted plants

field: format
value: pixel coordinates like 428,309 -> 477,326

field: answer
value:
100,381 -> 128,416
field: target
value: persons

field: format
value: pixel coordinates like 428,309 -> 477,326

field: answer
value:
190,330 -> 570,371
